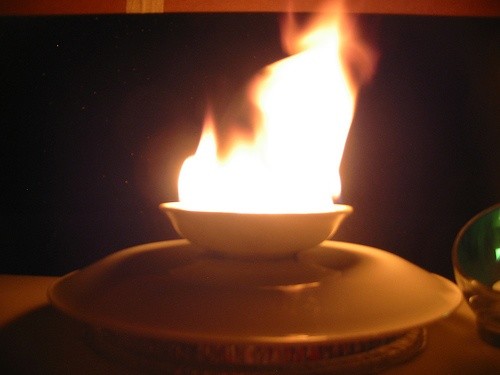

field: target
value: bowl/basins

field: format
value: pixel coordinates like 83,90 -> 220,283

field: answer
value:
456,204 -> 500,336
158,202 -> 353,257
